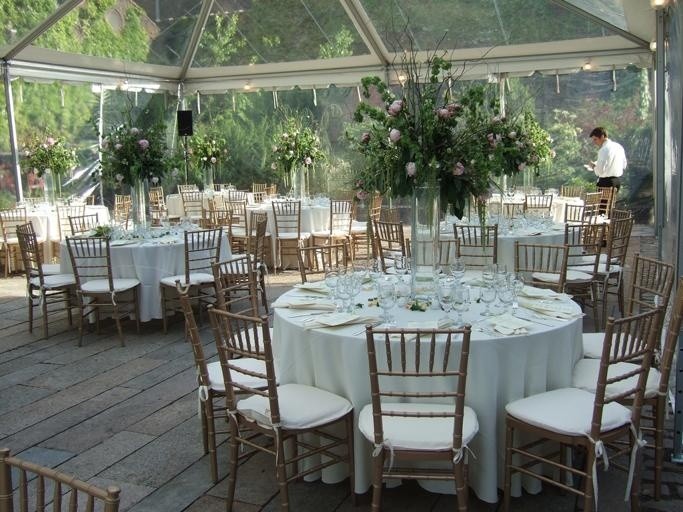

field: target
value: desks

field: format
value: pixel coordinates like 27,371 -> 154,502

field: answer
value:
485,191 -> 584,221
162,191 -> 267,217
238,200 -> 331,235
0,203 -> 110,263
414,222 -> 584,272
272,273 -> 582,500
58,231 -> 230,320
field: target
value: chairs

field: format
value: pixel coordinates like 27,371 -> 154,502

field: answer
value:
15,210 -> 270,348
476,186 -> 634,328
3,178 -> 383,272
175,238 -> 683,509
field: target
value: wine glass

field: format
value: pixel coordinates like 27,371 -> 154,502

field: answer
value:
321,255 -> 524,331
88,216 -> 199,246
473,208 -> 555,235
262,193 -> 330,210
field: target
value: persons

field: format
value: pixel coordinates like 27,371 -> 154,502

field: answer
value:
588,126 -> 628,248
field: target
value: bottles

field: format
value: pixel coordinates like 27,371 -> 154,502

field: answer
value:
444,204 -> 467,233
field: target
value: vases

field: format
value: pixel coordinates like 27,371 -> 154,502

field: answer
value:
131,168 -> 152,238
293,162 -> 307,200
43,166 -> 61,213
201,164 -> 214,193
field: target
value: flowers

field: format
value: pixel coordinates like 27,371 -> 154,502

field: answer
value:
345,20 -> 559,256
92,78 -> 177,185
178,130 -> 231,169
268,116 -> 328,177
19,126 -> 81,177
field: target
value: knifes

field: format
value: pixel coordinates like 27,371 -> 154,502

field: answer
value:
287,295 -> 323,298
289,310 -> 331,318
351,320 -> 380,336
512,314 -> 552,327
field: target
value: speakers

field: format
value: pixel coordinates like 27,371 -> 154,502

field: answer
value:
177,110 -> 192,136
